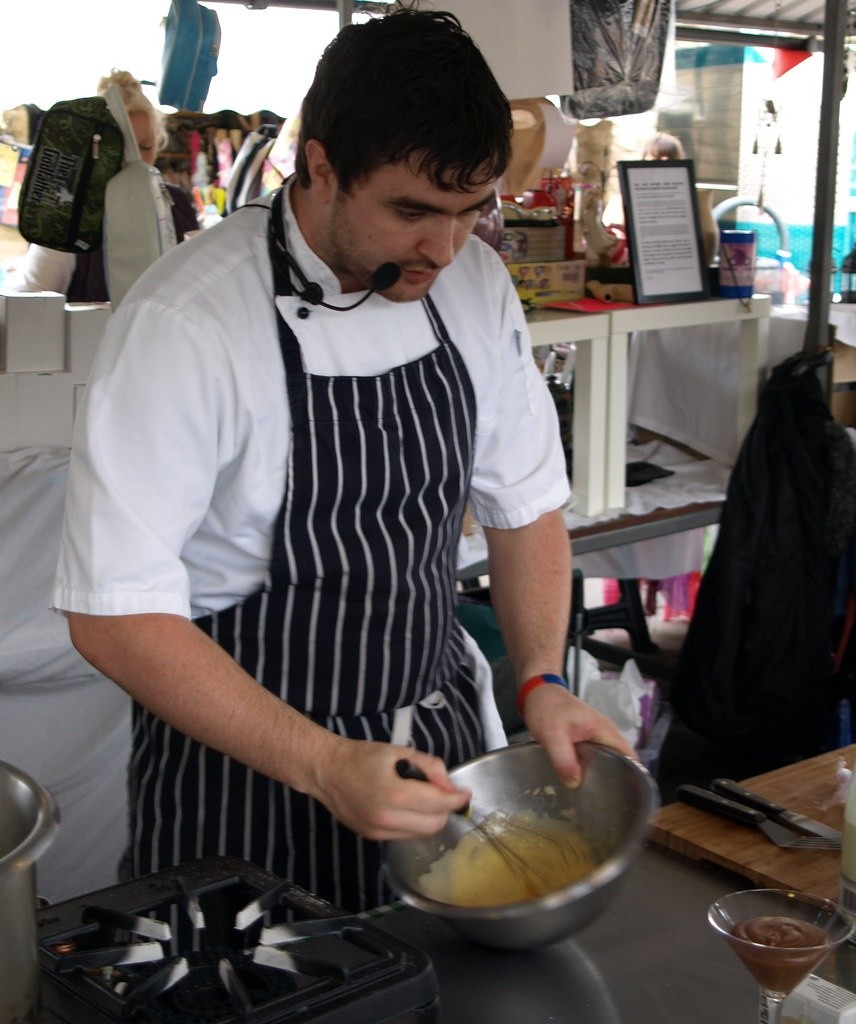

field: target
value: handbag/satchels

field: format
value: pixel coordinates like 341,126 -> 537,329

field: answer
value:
16,96 -> 124,254
103,86 -> 177,316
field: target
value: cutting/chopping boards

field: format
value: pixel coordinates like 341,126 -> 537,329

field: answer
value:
651,742 -> 856,910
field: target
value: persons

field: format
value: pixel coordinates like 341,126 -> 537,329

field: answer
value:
0,68 -> 216,303
52,4 -> 637,915
641,131 -> 684,161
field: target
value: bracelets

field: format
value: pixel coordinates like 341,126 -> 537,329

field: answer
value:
517,674 -> 570,719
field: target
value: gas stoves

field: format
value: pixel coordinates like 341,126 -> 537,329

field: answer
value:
39,855 -> 441,1024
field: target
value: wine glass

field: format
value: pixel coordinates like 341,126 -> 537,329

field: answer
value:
706,886 -> 856,1024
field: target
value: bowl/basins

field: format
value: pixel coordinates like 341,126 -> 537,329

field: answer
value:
379,742 -> 661,954
1,759 -> 62,1024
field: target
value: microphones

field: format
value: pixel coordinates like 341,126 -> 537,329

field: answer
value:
301,260 -> 401,311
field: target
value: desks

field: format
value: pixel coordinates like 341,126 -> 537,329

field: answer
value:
628,303 -> 856,465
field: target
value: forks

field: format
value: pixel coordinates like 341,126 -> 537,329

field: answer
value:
673,783 -> 841,852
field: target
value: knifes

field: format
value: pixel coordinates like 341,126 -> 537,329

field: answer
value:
715,777 -> 841,842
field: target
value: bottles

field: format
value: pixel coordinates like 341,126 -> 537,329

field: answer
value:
841,764 -> 856,918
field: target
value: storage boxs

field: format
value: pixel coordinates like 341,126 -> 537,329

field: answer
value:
504,219 -> 588,301
0,290 -> 112,454
